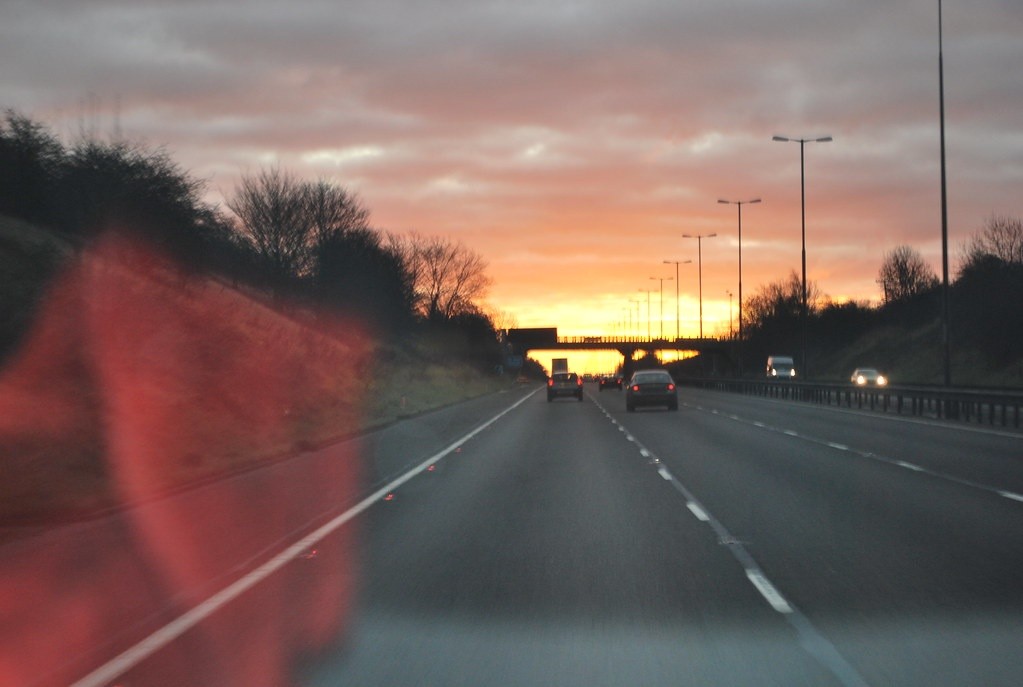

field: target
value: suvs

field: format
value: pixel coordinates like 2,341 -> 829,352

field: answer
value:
545,371 -> 583,403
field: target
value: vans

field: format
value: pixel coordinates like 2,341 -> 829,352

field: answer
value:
766,355 -> 796,381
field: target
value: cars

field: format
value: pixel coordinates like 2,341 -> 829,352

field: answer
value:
624,369 -> 678,413
579,373 -> 604,384
851,368 -> 888,389
597,374 -> 623,392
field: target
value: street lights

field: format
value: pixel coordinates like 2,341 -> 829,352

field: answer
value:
717,199 -> 763,377
662,261 -> 692,338
771,135 -> 832,383
649,276 -> 675,339
683,234 -> 719,338
